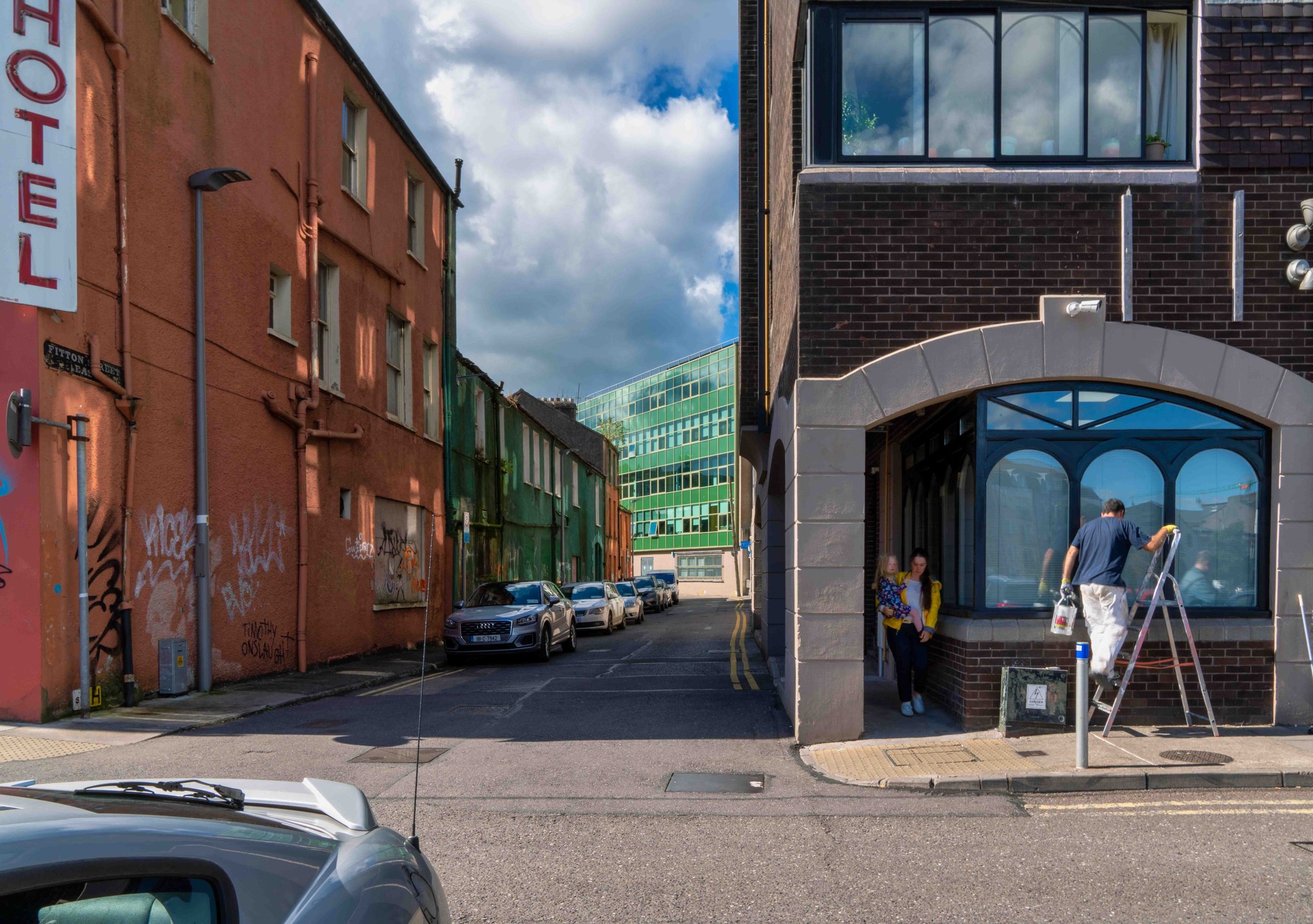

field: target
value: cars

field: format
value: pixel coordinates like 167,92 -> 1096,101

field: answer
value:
558,580 -> 626,633
656,580 -> 673,608
0,777 -> 452,924
611,581 -> 644,624
443,580 -> 577,664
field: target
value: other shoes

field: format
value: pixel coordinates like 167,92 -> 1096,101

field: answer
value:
1096,672 -> 1120,685
1088,671 -> 1114,691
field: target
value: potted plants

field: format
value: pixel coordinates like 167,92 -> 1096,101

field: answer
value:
1146,131 -> 1172,160
842,93 -> 879,154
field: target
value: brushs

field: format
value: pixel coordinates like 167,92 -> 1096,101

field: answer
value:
1064,588 -> 1080,604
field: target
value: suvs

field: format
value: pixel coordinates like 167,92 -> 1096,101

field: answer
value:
623,575 -> 665,613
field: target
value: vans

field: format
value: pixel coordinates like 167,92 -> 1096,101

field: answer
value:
646,569 -> 679,605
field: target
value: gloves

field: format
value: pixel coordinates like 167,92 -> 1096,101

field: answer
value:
1162,524 -> 1180,534
1038,580 -> 1049,597
1060,578 -> 1074,601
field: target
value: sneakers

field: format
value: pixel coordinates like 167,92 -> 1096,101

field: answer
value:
901,701 -> 914,717
913,695 -> 926,714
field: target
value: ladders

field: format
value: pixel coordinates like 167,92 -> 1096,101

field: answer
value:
1088,528 -> 1220,738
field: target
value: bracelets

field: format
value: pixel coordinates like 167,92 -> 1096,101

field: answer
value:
1041,578 -> 1048,580
1061,578 -> 1071,581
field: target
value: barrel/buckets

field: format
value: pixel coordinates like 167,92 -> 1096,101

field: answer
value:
1050,596 -> 1078,635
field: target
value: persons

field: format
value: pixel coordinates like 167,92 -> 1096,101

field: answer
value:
1060,497 -> 1179,691
872,548 -> 942,716
1038,503 -> 1090,600
1176,551 -> 1225,606
